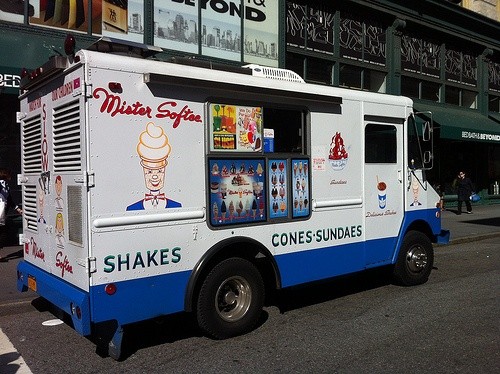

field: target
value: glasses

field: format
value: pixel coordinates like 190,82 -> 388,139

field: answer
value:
460,174 -> 463,175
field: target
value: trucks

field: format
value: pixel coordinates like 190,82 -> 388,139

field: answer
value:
12,32 -> 451,360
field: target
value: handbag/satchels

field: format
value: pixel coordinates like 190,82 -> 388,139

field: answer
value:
469,193 -> 481,202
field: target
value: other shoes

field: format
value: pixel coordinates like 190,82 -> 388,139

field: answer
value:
456,212 -> 461,215
467,210 -> 472,213
